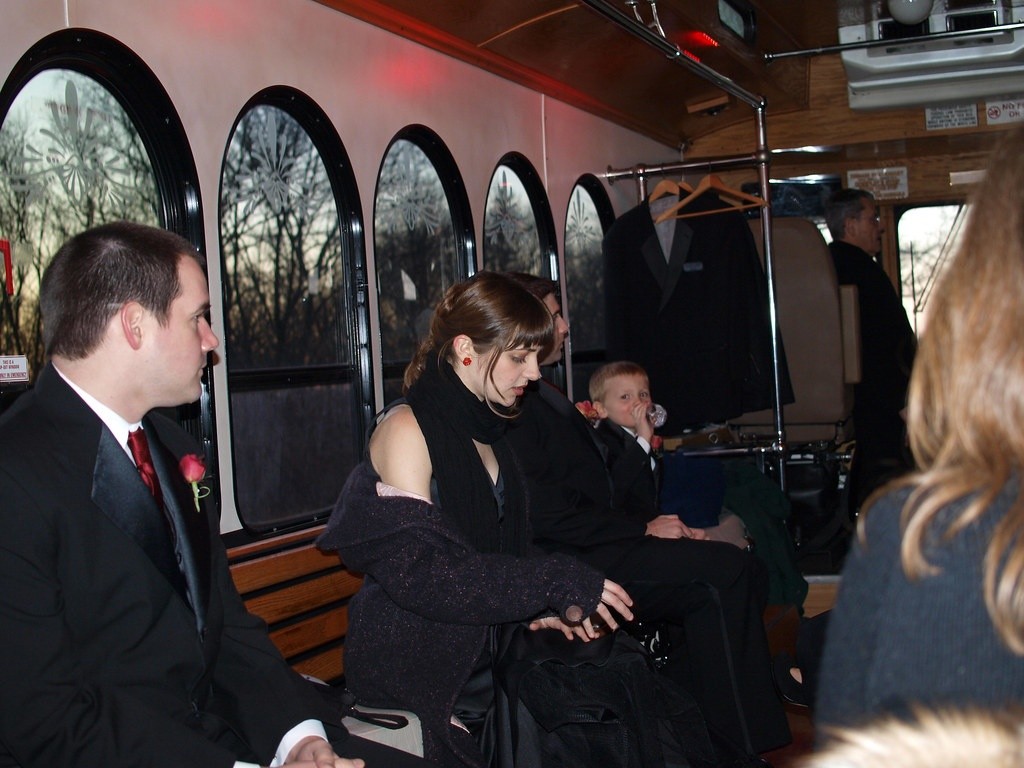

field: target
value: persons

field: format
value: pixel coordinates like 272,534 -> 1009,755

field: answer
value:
0,221 -> 437,768
313,270 -> 812,768
820,189 -> 919,470
794,120 -> 1024,767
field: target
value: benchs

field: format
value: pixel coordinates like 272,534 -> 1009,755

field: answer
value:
225,523 -> 366,688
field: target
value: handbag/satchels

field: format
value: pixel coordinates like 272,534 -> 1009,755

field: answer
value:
658,445 -> 728,528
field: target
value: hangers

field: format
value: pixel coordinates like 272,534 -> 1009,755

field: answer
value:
649,156 -> 767,223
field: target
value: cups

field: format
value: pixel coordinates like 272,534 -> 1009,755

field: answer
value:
644,404 -> 667,426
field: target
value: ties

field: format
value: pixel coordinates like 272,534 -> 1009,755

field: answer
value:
127,427 -> 164,517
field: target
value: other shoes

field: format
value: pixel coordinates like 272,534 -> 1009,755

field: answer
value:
769,653 -> 809,709
